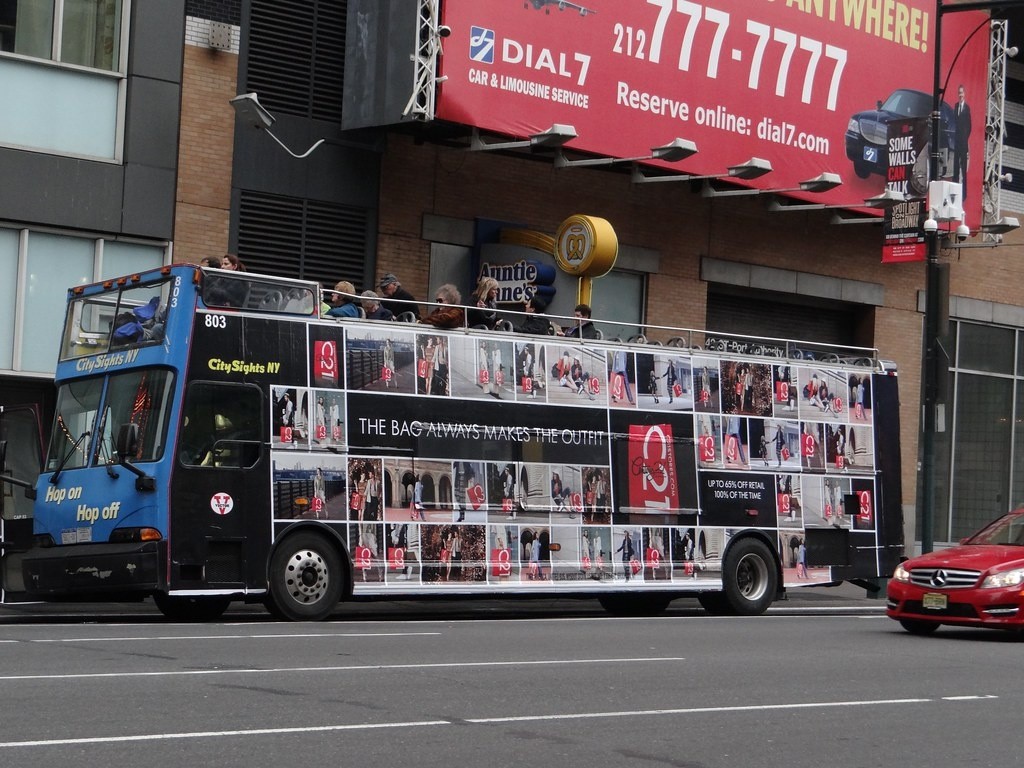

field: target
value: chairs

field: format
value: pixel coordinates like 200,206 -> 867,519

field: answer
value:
349,305 -> 878,367
200,414 -> 242,466
258,289 -> 285,311
280,289 -> 305,313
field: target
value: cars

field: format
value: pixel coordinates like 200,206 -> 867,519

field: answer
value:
845,89 -> 955,194
887,509 -> 1024,636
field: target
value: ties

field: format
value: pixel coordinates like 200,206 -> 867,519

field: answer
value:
959,104 -> 962,113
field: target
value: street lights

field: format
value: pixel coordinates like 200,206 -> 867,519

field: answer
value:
923,215 -> 1020,554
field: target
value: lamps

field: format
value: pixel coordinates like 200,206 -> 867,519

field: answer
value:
936,217 -> 1020,237
630,157 -> 773,183
437,24 -> 451,56
701,171 -> 842,199
465,124 -> 578,152
230,93 -> 325,159
766,189 -> 905,212
554,137 -> 698,168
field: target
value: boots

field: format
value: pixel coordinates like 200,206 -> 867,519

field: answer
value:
456,509 -> 465,522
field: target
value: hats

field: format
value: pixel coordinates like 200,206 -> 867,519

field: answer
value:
377,273 -> 397,287
813,373 -> 819,380
821,377 -> 827,385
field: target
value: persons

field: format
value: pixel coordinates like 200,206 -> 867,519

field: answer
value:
344,525 -> 809,585
305,272 -> 466,328
495,296 -> 549,335
556,304 -> 597,339
952,86 -> 972,204
269,333 -> 887,528
465,277 -> 503,329
150,254 -> 248,342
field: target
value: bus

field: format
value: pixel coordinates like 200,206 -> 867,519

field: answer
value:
21,262 -> 904,622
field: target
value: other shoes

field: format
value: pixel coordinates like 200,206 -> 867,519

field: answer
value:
654,398 -> 660,404
764,461 -> 769,466
824,404 -> 830,413
782,398 -> 795,412
513,512 -> 517,520
577,384 -> 584,394
526,394 -> 534,399
625,577 -> 629,583
631,401 -> 636,405
533,389 -> 537,399
611,396 -> 617,402
506,516 -> 513,520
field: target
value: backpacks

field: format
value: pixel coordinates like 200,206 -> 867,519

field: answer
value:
803,385 -> 811,397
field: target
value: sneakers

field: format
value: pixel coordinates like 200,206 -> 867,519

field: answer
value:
395,565 -> 412,580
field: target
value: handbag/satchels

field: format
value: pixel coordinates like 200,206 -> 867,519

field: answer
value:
700,433 -> 843,471
280,424 -> 341,443
777,490 -> 873,521
627,415 -> 680,508
382,367 -> 392,382
795,563 -> 804,578
832,397 -> 842,413
349,483 -> 694,579
314,340 -> 338,379
109,295 -> 167,343
312,497 -> 322,512
855,402 -> 863,418
417,359 -> 788,403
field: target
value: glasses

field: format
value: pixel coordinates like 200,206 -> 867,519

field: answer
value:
435,298 -> 443,303
